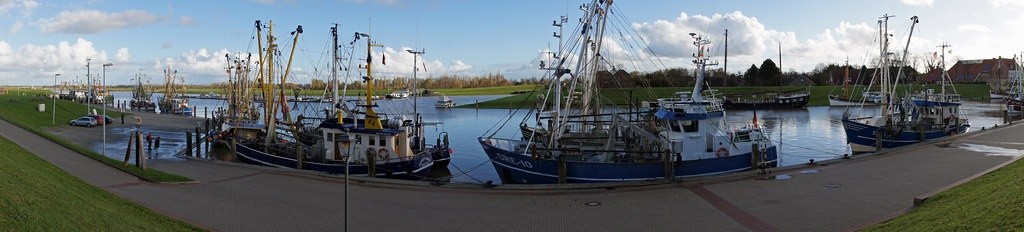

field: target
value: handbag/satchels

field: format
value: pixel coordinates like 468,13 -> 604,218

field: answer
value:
154,147 -> 158,150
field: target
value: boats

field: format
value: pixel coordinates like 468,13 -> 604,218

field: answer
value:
433,93 -> 456,108
723,82 -> 811,110
384,91 -> 410,99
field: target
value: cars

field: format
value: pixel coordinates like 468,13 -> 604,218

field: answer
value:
102,115 -> 114,125
86,115 -> 103,125
70,117 -> 98,128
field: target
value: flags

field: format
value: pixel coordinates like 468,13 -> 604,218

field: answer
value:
753,103 -> 757,128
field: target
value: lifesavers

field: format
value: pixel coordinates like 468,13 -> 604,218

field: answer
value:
377,149 -> 390,159
716,148 -> 729,160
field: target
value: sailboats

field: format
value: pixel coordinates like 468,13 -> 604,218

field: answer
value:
988,55 -> 1016,99
477,0 -> 778,184
157,64 -> 193,116
128,70 -> 157,113
827,20 -> 907,108
1001,51 -> 1024,120
207,19 -> 451,179
840,15 -> 972,157
60,73 -> 114,106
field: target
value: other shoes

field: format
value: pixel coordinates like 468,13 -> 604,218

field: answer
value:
148,149 -> 152,150
156,153 -> 159,155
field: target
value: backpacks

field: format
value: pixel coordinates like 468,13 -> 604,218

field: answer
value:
146,136 -> 150,141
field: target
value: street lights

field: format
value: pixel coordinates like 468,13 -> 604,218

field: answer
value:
102,63 -> 114,155
86,59 -> 90,116
53,73 -> 60,124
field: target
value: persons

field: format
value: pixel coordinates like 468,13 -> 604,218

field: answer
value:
154,136 -> 161,155
121,113 -> 125,124
146,132 -> 154,151
